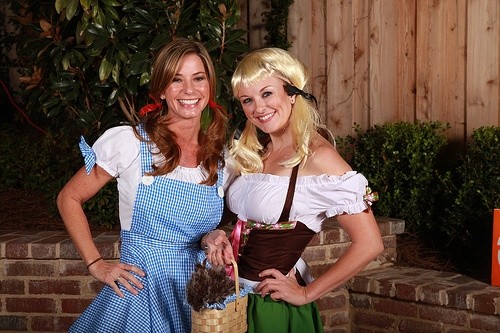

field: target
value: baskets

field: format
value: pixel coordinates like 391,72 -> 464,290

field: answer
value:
190,254 -> 248,333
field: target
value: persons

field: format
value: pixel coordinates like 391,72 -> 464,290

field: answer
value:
225,47 -> 384,333
57,39 -> 241,333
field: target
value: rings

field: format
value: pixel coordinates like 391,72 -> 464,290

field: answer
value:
116,276 -> 121,280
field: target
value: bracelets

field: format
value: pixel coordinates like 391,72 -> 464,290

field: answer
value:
87,257 -> 103,268
203,230 -> 214,248
